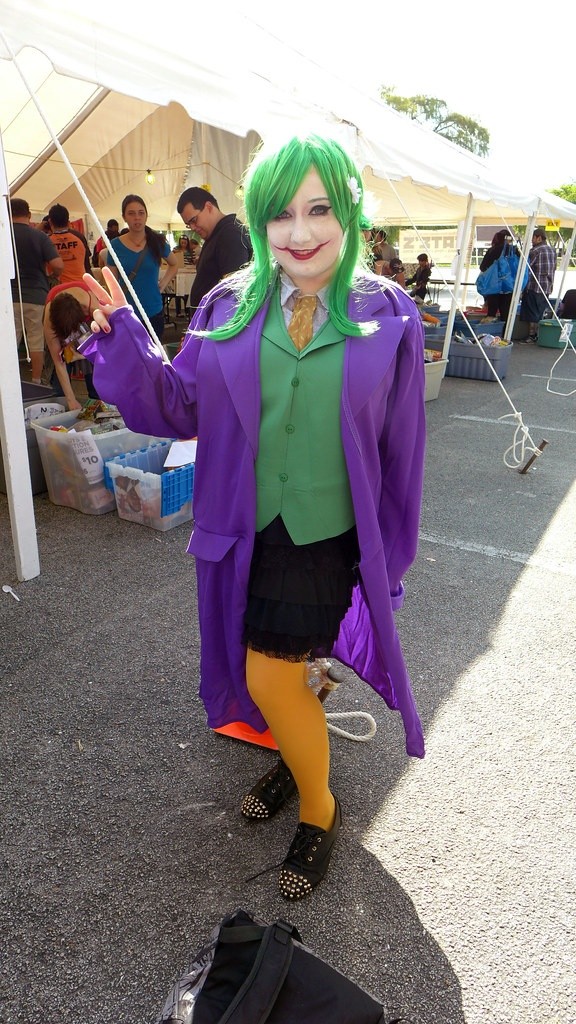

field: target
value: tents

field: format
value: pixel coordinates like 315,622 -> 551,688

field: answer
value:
233,0 -> 575,347
0,0 -> 550,580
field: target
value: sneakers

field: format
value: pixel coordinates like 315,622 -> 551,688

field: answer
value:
519,336 -> 538,344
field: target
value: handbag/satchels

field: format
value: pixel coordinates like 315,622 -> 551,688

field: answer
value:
476,239 -> 530,295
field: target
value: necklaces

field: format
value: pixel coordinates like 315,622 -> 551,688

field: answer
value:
128,235 -> 145,246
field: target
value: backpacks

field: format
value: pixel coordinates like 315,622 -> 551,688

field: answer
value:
156,907 -> 385,1024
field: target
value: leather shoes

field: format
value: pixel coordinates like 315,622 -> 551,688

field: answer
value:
241,756 -> 298,822
246,792 -> 341,901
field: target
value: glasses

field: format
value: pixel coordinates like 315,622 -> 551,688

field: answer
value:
180,235 -> 187,239
185,203 -> 206,229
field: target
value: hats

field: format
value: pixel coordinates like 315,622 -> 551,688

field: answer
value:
390,258 -> 404,273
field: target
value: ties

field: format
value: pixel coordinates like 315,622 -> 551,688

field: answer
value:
288,291 -> 317,352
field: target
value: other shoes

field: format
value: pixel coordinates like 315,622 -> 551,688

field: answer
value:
176,314 -> 185,318
39,377 -> 47,386
187,313 -> 190,316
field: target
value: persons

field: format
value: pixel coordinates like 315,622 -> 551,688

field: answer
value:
190,239 -> 203,256
96,219 -> 128,267
49,205 -> 96,283
178,187 -> 253,322
479,230 -> 520,321
42,281 -> 101,411
405,254 -> 430,299
174,235 -> 195,318
107,195 -> 178,347
365,228 -> 404,287
519,229 -> 557,344
7,199 -> 63,386
83,133 -> 426,904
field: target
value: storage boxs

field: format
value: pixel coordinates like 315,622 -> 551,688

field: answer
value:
419,298 -> 576,401
0,379 -> 201,533
